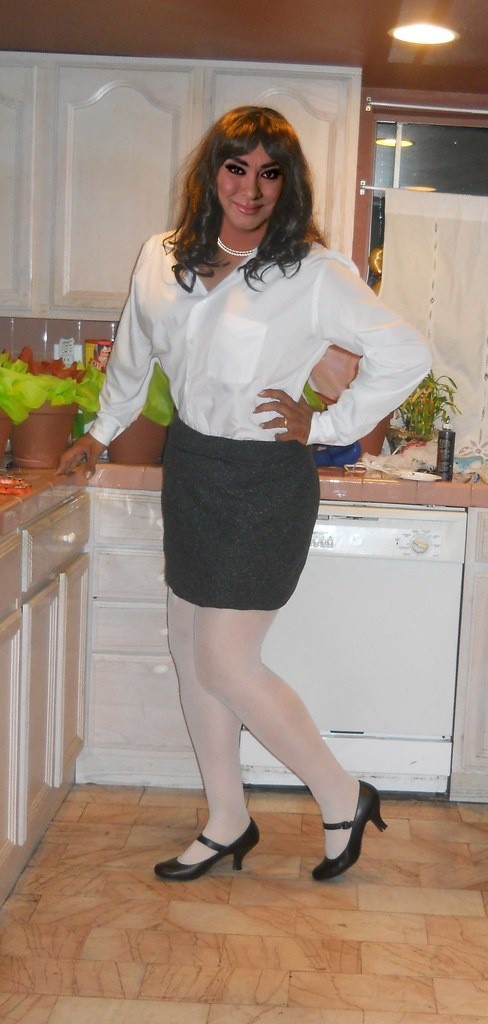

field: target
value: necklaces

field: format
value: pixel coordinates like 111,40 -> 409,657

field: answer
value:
217,236 -> 257,256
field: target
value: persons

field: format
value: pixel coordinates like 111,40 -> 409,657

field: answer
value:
56,106 -> 436,882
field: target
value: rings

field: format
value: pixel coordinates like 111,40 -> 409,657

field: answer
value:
284,418 -> 288,428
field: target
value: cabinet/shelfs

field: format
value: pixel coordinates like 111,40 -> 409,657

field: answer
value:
0,489 -> 90,906
1,52 -> 363,321
447,507 -> 488,803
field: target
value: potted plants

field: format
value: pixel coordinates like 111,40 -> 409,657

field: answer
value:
388,370 -> 462,456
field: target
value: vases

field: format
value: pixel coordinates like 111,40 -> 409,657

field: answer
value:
107,413 -> 170,463
11,402 -> 78,469
355,409 -> 396,461
0,408 -> 12,465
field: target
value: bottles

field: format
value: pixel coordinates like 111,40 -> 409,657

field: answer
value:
435,425 -> 455,481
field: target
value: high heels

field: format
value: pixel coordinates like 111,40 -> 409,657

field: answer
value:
312,780 -> 388,881
154,817 -> 260,881
